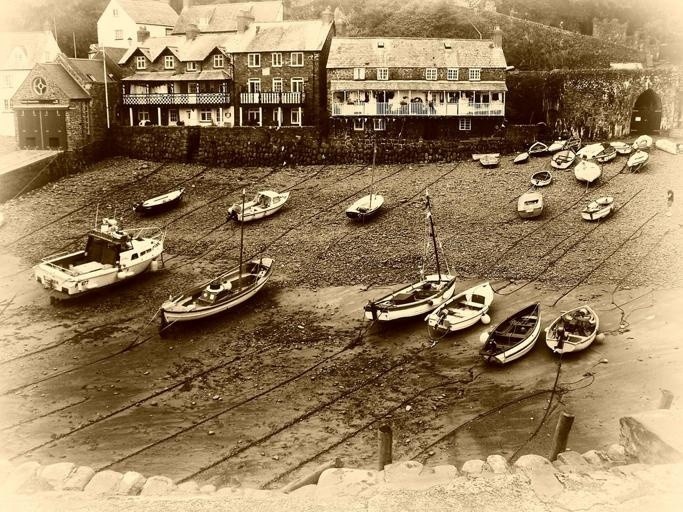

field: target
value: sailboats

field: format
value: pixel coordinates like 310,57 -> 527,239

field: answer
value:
345,138 -> 384,220
362,186 -> 457,329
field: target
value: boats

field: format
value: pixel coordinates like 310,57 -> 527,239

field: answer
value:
141,185 -> 187,211
159,256 -> 277,325
471,135 -> 683,222
424,281 -> 601,366
228,189 -> 292,224
30,201 -> 169,298
